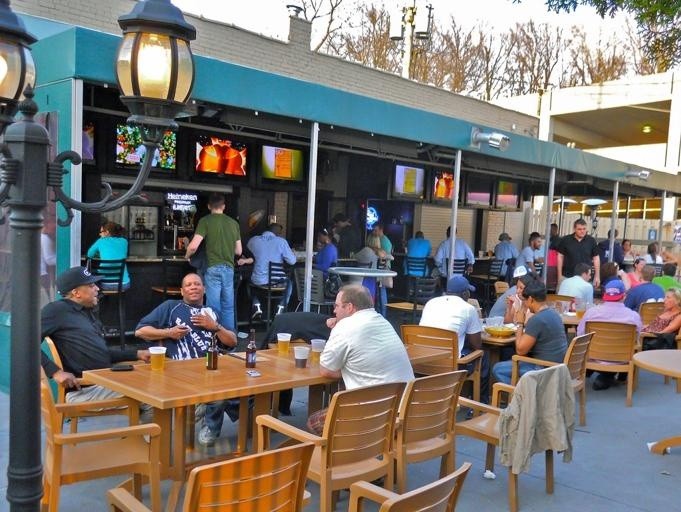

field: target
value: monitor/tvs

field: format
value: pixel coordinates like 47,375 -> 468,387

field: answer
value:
111,365 -> 134,371
395,165 -> 424,198
82,121 -> 96,165
498,181 -> 520,210
195,133 -> 246,176
115,122 -> 176,169
467,174 -> 493,205
434,173 -> 454,199
261,145 -> 303,182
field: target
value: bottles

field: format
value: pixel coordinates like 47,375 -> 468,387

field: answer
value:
205,328 -> 257,371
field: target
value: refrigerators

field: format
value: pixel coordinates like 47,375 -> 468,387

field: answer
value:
99,188 -> 163,259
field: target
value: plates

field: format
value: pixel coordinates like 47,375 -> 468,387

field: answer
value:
484,327 -> 514,338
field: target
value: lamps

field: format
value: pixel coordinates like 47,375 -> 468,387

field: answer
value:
626,167 -> 653,183
471,124 -> 512,156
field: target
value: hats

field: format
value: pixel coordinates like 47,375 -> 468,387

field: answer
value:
447,274 -> 476,293
499,233 -> 512,241
602,279 -> 625,301
56,266 -> 103,295
513,265 -> 533,278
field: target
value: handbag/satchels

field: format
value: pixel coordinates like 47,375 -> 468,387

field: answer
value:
323,275 -> 343,299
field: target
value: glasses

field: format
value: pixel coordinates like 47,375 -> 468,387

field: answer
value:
332,303 -> 349,308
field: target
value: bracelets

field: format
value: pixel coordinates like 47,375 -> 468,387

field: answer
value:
213,323 -> 223,334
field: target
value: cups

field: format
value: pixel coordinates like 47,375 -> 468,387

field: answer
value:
147,345 -> 167,372
575,296 -> 587,318
478,316 -> 504,330
479,249 -> 494,259
275,332 -> 325,370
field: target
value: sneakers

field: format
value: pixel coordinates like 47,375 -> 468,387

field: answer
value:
195,402 -> 208,423
464,407 -> 474,419
251,303 -> 263,321
272,304 -> 286,318
198,424 -> 217,447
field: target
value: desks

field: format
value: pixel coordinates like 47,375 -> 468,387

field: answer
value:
633,346 -> 681,454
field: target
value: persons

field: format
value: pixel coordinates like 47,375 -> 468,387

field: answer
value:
548,218 -> 678,292
86,221 -> 131,305
38,266 -> 154,425
558,258 -> 680,391
401,224 -> 475,288
246,221 -> 298,322
488,231 -> 568,396
39,226 -> 56,295
182,193 -> 243,331
305,283 -> 416,490
419,275 -> 489,393
234,230 -> 261,270
311,213 -> 394,318
134,272 -> 238,447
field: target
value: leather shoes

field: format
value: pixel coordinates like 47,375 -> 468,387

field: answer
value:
279,407 -> 291,416
